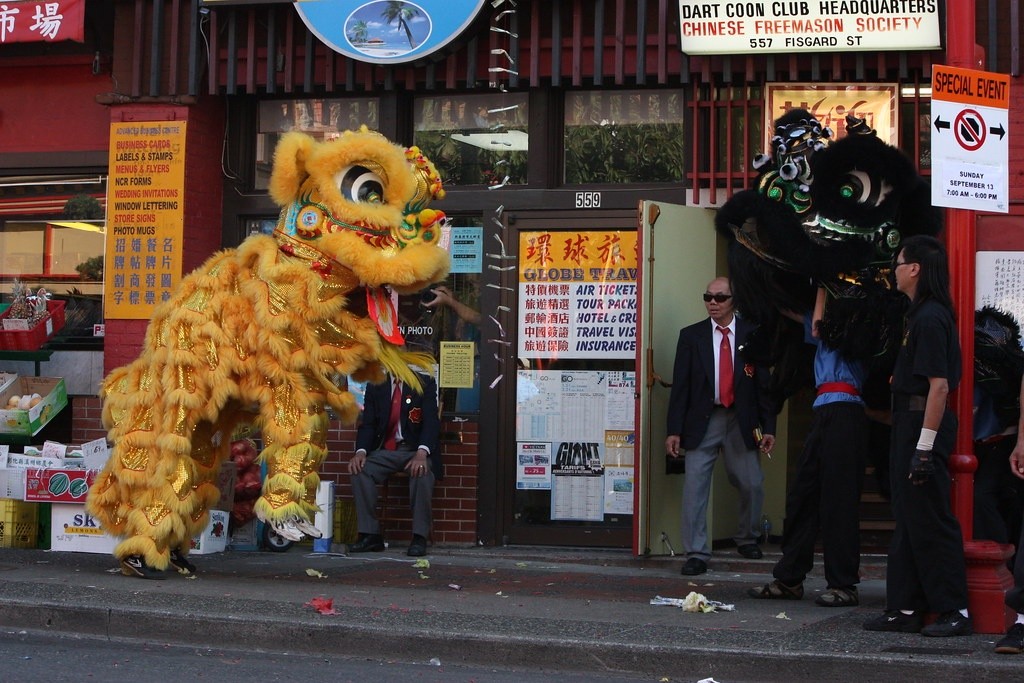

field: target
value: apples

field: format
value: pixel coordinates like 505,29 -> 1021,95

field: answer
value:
4,394 -> 42,411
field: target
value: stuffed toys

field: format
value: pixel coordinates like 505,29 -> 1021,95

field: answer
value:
84,124 -> 450,580
712,108 -> 1024,542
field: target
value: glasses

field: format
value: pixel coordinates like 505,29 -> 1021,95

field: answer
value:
703,294 -> 732,303
894,262 -> 913,270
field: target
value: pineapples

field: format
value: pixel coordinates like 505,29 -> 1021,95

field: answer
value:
10,279 -> 52,328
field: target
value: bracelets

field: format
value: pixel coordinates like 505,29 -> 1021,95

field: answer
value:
916,428 -> 937,451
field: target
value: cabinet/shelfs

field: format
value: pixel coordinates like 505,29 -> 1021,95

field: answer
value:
0,350 -> 73,455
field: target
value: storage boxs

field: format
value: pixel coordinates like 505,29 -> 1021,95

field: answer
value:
0,299 -> 66,349
0,376 -> 68,436
299,497 -> 355,546
50,504 -> 125,555
24,467 -> 96,504
37,503 -> 51,549
0,499 -> 38,549
188,509 -> 229,555
8,452 -> 86,470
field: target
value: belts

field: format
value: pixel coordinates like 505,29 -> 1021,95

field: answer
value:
385,378 -> 402,450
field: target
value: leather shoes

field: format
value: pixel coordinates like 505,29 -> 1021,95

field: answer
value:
349,535 -> 385,552
407,534 -> 427,556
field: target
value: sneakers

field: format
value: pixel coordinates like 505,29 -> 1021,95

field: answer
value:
124,554 -> 169,579
993,623 -> 1024,654
864,609 -> 918,633
749,579 -> 804,600
256,510 -> 309,542
682,559 -> 708,575
292,515 -> 322,539
169,549 -> 196,572
737,543 -> 763,559
814,585 -> 859,607
919,610 -> 973,637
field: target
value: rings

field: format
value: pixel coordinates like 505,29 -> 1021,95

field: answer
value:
420,465 -> 423,467
666,447 -> 669,451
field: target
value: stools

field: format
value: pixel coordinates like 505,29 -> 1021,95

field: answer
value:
381,471 -> 435,548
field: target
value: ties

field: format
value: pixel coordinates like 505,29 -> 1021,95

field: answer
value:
716,326 -> 735,408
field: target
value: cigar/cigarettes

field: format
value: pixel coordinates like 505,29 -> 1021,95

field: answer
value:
764,447 -> 772,459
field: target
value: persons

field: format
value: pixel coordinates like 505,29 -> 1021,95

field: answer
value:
348,367 -> 442,556
994,373 -> 1024,653
666,276 -> 777,576
421,271 -> 483,414
863,234 -> 973,636
746,284 -> 868,606
